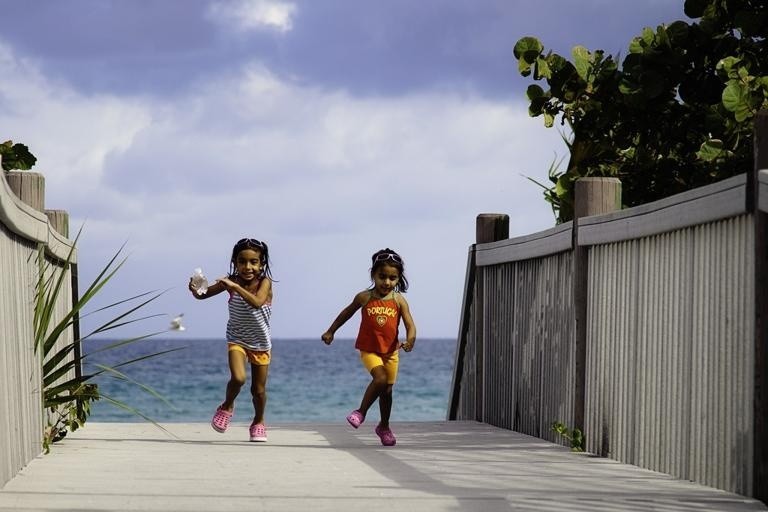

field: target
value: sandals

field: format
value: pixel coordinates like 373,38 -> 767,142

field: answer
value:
346,408 -> 365,428
211,403 -> 234,433
375,425 -> 398,446
249,423 -> 268,443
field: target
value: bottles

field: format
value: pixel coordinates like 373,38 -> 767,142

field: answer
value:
192,267 -> 209,295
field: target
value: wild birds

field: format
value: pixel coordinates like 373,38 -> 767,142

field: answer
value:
170,313 -> 186,331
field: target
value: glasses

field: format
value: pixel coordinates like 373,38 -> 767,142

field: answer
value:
372,253 -> 403,265
235,238 -> 263,249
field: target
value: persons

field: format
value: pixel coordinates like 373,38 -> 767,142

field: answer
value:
321,246 -> 417,447
188,238 -> 275,443
